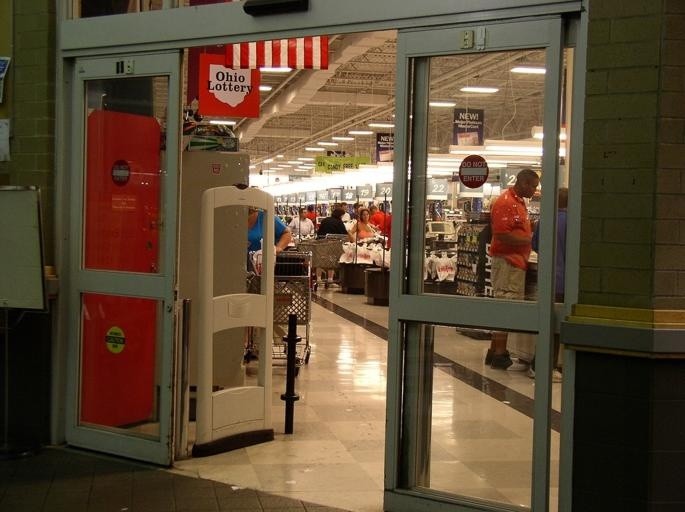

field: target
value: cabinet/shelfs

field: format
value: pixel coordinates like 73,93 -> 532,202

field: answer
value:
451,221 -> 493,341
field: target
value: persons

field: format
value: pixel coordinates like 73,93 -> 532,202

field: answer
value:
487,169 -> 540,368
243,204 -> 291,363
530,187 -> 568,383
289,202 -> 393,288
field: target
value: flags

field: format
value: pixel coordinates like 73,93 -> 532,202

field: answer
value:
197,53 -> 262,119
224,35 -> 329,71
315,108 -> 484,174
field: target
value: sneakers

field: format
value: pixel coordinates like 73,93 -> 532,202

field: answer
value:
485,348 -> 520,364
491,352 -> 528,371
317,280 -> 341,288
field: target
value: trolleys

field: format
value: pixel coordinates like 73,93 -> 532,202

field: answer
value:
244,249 -> 318,378
295,229 -> 352,295
